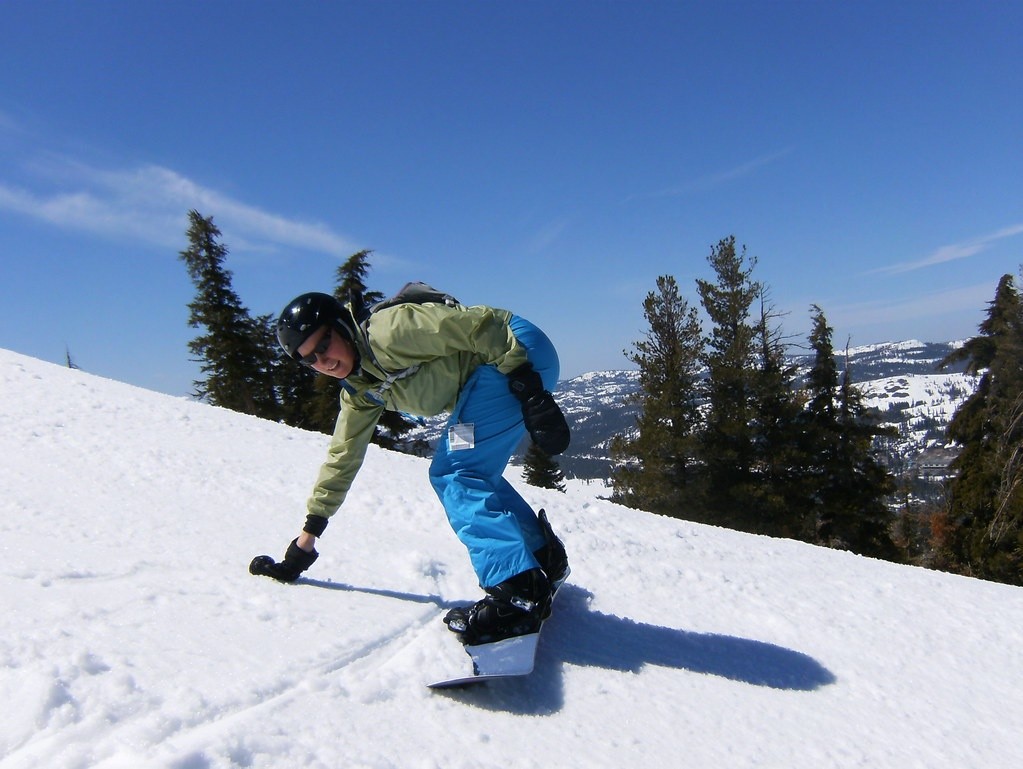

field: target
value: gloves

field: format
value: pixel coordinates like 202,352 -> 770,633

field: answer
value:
249,536 -> 319,582
508,362 -> 570,456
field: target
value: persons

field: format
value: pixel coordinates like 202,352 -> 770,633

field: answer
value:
249,280 -> 570,645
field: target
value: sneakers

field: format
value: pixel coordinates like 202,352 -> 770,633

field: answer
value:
533,508 -> 571,580
443,568 -> 554,646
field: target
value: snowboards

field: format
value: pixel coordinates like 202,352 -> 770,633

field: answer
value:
429,560 -> 569,690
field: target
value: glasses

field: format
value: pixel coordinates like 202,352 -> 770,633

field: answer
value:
299,324 -> 333,365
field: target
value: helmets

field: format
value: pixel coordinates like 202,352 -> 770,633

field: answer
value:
277,292 -> 358,359
